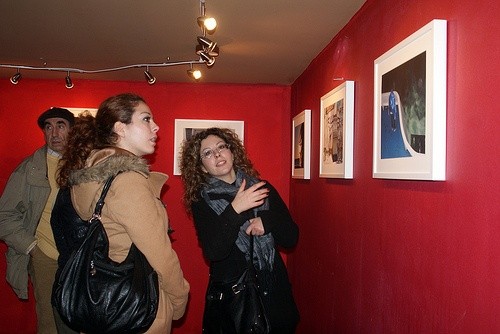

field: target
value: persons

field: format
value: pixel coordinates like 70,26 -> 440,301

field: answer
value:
51,185 -> 82,334
180,128 -> 301,334
0,107 -> 75,334
56,93 -> 190,334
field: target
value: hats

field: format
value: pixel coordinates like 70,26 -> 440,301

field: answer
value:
38,108 -> 75,127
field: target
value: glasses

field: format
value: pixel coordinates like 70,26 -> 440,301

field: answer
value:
199,141 -> 229,161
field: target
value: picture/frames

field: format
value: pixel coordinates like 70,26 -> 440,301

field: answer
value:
319,80 -> 355,180
174,119 -> 245,176
291,110 -> 312,180
372,19 -> 448,180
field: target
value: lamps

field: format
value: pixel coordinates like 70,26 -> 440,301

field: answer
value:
197,5 -> 216,30
195,36 -> 219,66
144,66 -> 156,84
65,71 -> 74,88
188,63 -> 201,79
12,67 -> 21,84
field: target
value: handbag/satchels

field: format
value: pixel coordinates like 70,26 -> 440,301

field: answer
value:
228,234 -> 300,334
51,170 -> 160,334
